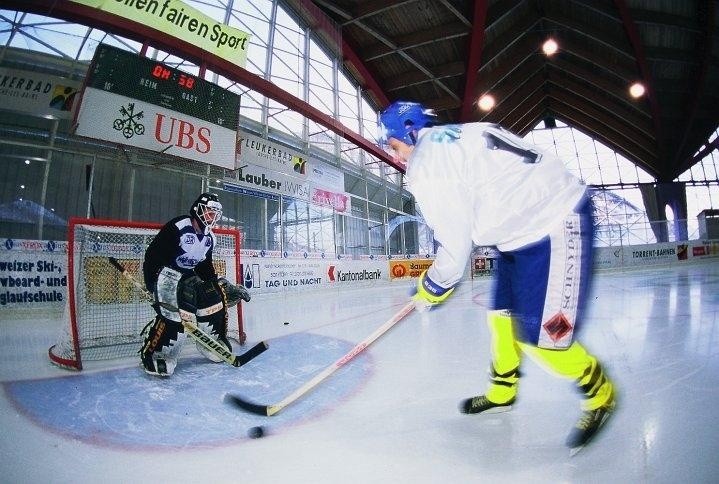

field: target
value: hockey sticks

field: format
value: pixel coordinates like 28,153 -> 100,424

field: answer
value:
109,257 -> 269,367
223,301 -> 414,417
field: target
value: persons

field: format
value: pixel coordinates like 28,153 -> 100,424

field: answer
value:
138,192 -> 251,377
378,101 -> 615,449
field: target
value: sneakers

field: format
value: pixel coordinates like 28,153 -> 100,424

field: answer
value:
571,396 -> 613,442
464,395 -> 516,414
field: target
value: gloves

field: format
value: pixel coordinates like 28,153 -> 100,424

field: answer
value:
409,269 -> 460,313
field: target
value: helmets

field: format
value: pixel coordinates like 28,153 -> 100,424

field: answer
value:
379,103 -> 434,166
189,193 -> 223,236
